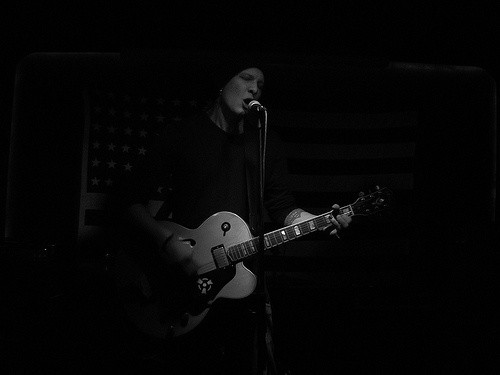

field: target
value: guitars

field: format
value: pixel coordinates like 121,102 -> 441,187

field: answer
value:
106,184 -> 392,340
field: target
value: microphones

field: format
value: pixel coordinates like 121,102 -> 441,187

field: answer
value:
247,100 -> 266,113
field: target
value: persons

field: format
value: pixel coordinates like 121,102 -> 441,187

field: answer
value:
114,51 -> 353,375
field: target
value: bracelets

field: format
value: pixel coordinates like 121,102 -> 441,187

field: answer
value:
161,234 -> 174,251
